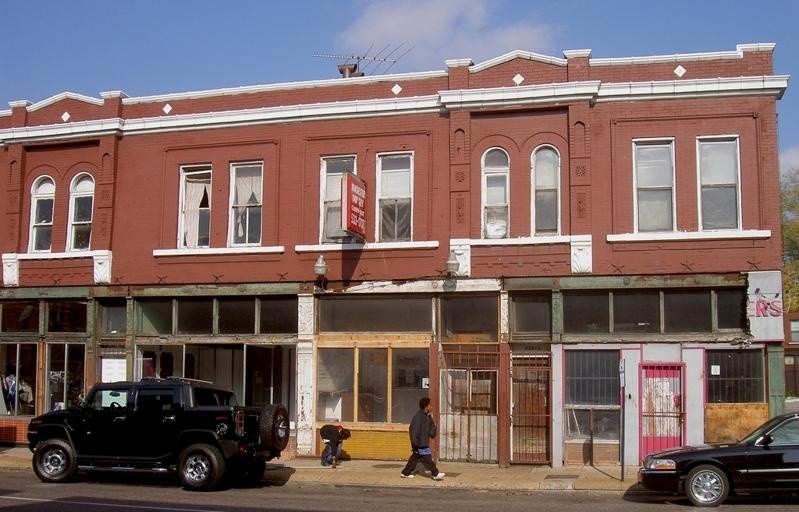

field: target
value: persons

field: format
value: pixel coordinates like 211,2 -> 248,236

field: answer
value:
319,424 -> 352,468
398,397 -> 447,481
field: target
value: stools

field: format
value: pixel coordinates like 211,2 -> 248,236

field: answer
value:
23,403 -> 34,415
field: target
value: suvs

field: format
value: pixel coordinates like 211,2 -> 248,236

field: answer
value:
26,376 -> 290,491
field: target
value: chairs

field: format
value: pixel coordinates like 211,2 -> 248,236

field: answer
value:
131,396 -> 164,429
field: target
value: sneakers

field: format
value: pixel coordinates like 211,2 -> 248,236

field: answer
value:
432,472 -> 446,480
400,472 -> 415,479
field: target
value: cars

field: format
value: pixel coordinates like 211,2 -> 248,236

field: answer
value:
638,411 -> 799,507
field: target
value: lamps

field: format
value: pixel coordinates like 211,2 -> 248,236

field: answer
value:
313,254 -> 327,276
446,249 -> 459,277
